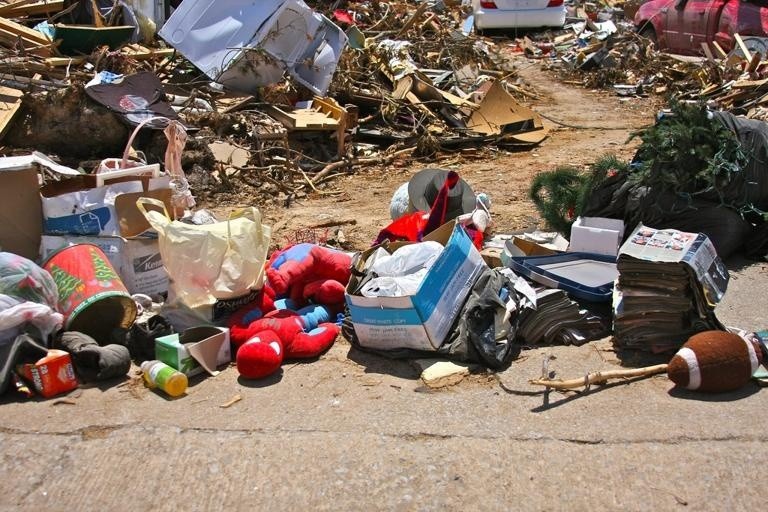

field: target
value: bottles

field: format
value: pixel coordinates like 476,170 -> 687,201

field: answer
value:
139,358 -> 190,396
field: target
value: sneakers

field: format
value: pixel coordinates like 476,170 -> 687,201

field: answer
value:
51,330 -> 132,384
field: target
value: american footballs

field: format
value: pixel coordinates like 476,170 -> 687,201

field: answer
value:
668,331 -> 763,393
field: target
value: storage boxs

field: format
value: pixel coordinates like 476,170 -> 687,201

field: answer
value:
1,167 -> 176,297
349,219 -> 488,352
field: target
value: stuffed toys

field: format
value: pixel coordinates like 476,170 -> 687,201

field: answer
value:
226,240 -> 353,381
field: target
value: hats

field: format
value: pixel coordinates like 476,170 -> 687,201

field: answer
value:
406,167 -> 477,225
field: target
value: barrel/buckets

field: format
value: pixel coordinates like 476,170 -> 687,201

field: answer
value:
36,239 -> 138,344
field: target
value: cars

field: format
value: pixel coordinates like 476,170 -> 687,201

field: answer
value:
471,0 -> 566,37
635,0 -> 768,57
